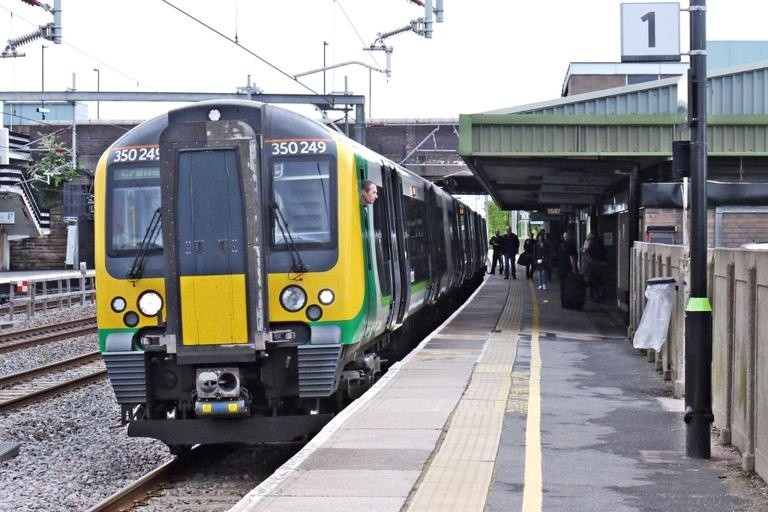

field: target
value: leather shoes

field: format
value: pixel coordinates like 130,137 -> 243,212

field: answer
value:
487,272 -> 534,280
591,297 -> 604,303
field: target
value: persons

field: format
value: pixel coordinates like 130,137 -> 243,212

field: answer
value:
360,180 -> 378,204
487,226 -> 577,290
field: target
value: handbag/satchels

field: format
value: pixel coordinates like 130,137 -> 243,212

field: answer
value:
518,251 -> 529,266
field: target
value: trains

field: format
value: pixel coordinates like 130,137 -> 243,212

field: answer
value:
93,99 -> 488,459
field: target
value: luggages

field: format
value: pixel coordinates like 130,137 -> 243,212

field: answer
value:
561,270 -> 585,309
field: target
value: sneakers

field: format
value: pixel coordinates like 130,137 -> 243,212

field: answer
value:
543,284 -> 546,290
538,286 -> 543,289
